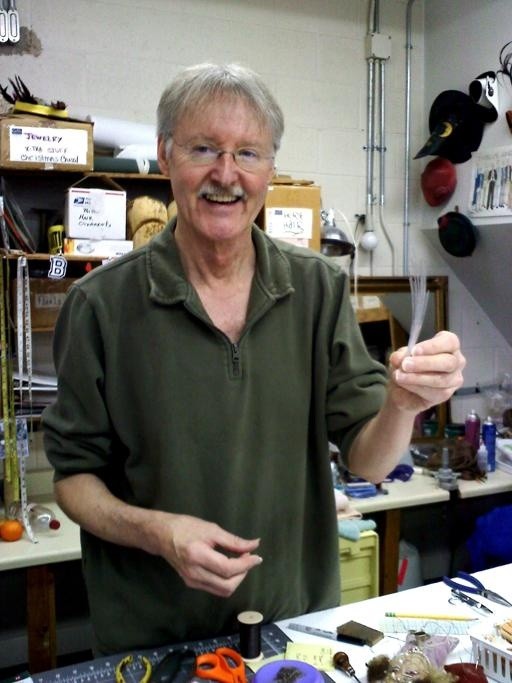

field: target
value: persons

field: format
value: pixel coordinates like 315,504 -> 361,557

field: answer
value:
41,62 -> 468,663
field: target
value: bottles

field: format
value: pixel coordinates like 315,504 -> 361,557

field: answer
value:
482,416 -> 495,470
477,442 -> 487,471
6,500 -> 61,531
464,408 -> 480,451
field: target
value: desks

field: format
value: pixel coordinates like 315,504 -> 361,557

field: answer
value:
1,430 -> 511,576
0,561 -> 512,682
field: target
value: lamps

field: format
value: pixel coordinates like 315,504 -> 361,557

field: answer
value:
319,207 -> 359,274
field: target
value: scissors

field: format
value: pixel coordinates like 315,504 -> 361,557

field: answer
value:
195,646 -> 247,683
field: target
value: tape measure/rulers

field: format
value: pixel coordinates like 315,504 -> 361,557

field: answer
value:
18,256 -> 39,543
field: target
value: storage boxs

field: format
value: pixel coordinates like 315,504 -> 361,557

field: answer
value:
63,174 -> 128,240
0,113 -> 95,172
264,183 -> 323,255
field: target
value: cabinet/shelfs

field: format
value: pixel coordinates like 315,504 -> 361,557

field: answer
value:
0,164 -> 172,341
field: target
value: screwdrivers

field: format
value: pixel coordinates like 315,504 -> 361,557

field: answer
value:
333,652 -> 361,683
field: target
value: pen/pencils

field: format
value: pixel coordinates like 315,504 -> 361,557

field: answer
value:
385,612 -> 479,621
288,623 -> 338,640
451,589 -> 494,614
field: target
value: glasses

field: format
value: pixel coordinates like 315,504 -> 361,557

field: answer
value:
170,136 -> 273,171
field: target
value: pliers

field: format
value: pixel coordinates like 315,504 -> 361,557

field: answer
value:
443,571 -> 512,607
116,656 -> 151,683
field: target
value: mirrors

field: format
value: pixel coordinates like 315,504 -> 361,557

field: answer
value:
346,275 -> 452,442
